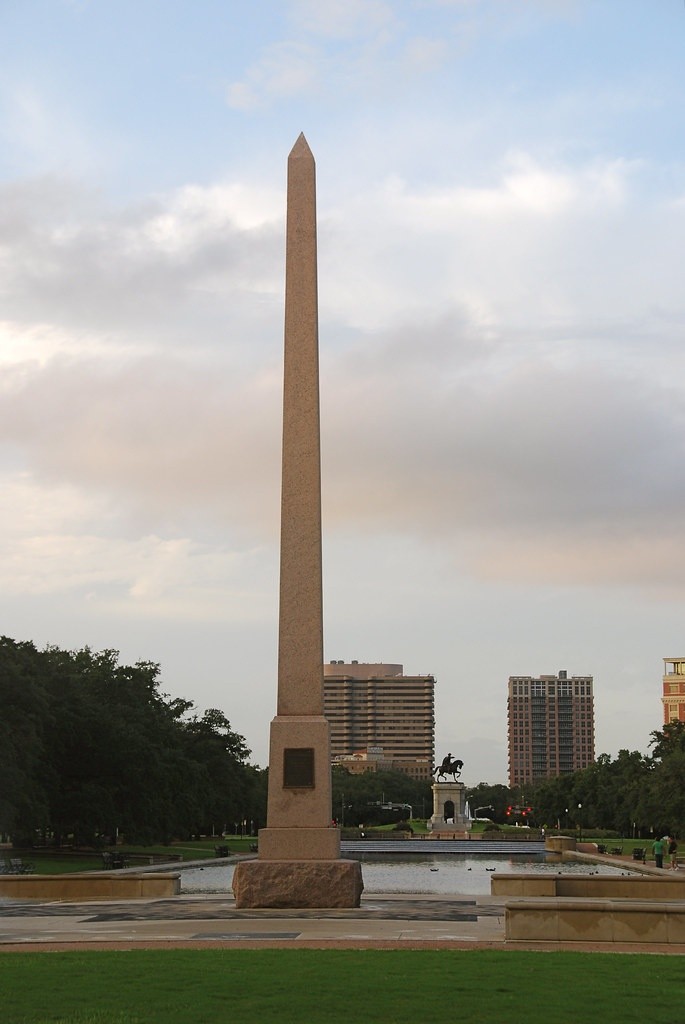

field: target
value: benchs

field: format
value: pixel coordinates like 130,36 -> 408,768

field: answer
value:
611,845 -> 623,855
598,844 -> 608,854
215,843 -> 229,857
102,851 -> 130,870
248,840 -> 258,852
632,845 -> 647,859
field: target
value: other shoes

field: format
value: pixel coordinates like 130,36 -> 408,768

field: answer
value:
675,867 -> 680,871
668,868 -> 674,871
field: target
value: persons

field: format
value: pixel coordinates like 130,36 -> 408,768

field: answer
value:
540,827 -> 546,839
652,837 -> 666,868
667,837 -> 680,870
445,753 -> 454,774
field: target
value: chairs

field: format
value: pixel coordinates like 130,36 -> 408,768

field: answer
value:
0,858 -> 35,875
